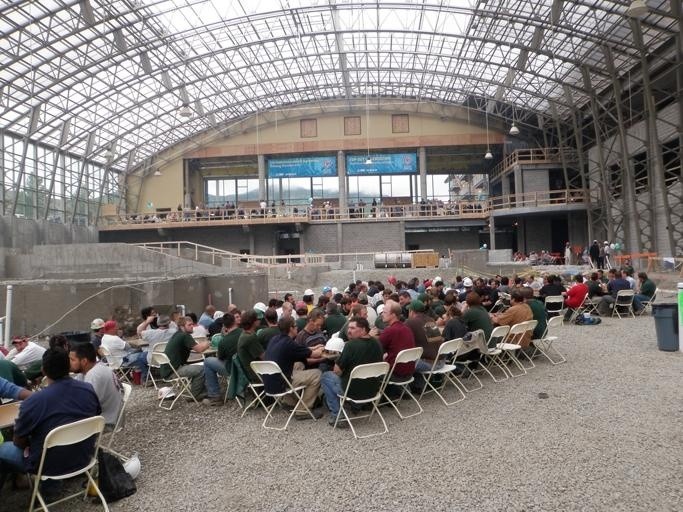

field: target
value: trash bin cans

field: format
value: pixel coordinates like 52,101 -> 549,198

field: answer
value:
652,306 -> 676,351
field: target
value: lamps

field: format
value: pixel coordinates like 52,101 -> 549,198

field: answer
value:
484,89 -> 493,160
153,139 -> 161,177
625,1 -> 650,17
180,101 -> 194,116
510,95 -> 520,134
365,88 -> 373,167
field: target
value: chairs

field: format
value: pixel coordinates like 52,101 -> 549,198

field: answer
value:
28,417 -> 111,512
100,346 -> 134,386
496,322 -> 528,377
641,288 -> 659,313
250,361 -> 317,431
525,318 -> 537,333
331,362 -> 390,440
568,292 -> 590,324
612,290 -> 636,319
144,343 -> 170,390
546,296 -> 565,332
580,293 -> 602,316
448,330 -> 486,392
185,341 -> 221,381
96,384 -> 131,479
152,353 -> 200,410
367,346 -> 425,421
419,337 -> 465,407
232,354 -> 273,419
212,354 -> 243,409
469,325 -> 511,383
534,316 -> 566,364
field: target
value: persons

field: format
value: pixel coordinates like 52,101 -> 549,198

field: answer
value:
268,298 -> 280,312
432,294 -> 457,321
356,198 -> 367,219
295,313 -> 327,346
321,285 -> 333,300
251,300 -> 270,314
270,198 -> 336,220
453,275 -> 465,290
495,274 -> 502,280
0,358 -> 28,388
203,313 -> 243,407
490,280 -> 501,301
120,213 -> 161,223
378,284 -> 386,292
511,277 -> 531,299
259,199 -> 268,218
394,283 -> 406,295
520,284 -> 549,341
278,299 -> 285,308
403,297 -> 447,396
166,201 -> 247,221
139,306 -> 160,332
538,273 -> 567,320
618,259 -> 635,277
168,310 -> 182,323
302,288 -> 317,316
323,302 -> 347,340
308,295 -> 331,320
0,345 -> 106,509
461,290 -> 498,367
197,304 -> 217,330
355,279 -> 363,293
621,270 -> 637,290
212,310 -> 225,321
411,276 -> 420,286
416,285 -> 426,295
458,277 -> 474,303
423,278 -> 430,284
388,292 -> 400,303
69,342 -> 126,436
333,292 -> 344,304
183,312 -> 209,339
603,240 -> 613,270
432,275 -> 443,286
397,291 -> 414,321
404,279 -> 417,298
511,248 -> 560,263
497,275 -> 513,294
434,280 -> 447,300
438,304 -> 471,362
294,300 -> 311,332
597,269 -> 609,284
90,317 -> 106,360
357,292 -> 378,331
338,303 -> 369,342
472,277 -> 489,296
284,291 -> 296,310
227,303 -> 238,313
320,315 -> 384,427
374,280 -> 382,288
230,308 -> 242,325
256,308 -> 282,352
607,268 -> 618,285
348,283 -> 356,294
560,274 -> 590,321
479,243 -> 488,251
420,196 -> 484,216
368,280 -> 375,288
490,289 -> 535,352
564,241 -> 572,266
589,239 -> 600,268
370,197 -> 378,218
48,333 -> 68,352
261,316 -> 325,420
376,304 -> 386,317
278,301 -> 297,329
161,315 -> 211,401
236,310 -> 267,408
583,272 -> 603,304
136,314 -> 177,383
4,334 -> 47,387
552,276 -> 568,310
357,284 -> 368,301
382,288 -> 393,303
597,241 -> 605,269
632,272 -> 657,316
597,271 -> 631,316
101,319 -> 148,385
0,376 -> 34,401
525,274 -> 542,291
375,299 -> 417,409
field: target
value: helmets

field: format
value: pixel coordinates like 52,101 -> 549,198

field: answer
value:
90,319 -> 105,329
304,289 -> 315,295
593,240 -> 597,242
377,304 -> 384,314
463,278 -> 473,287
82,476 -> 100,497
323,287 -> 332,294
157,387 -> 176,400
325,337 -> 344,352
254,302 -> 267,313
157,315 -> 171,326
604,241 -> 608,244
122,453 -> 141,479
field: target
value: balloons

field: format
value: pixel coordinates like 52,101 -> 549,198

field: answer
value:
614,242 -> 622,250
609,242 -> 615,250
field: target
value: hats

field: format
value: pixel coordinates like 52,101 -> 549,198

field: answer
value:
387,275 -> 396,285
405,300 -> 425,311
425,282 -> 432,288
12,335 -> 28,344
103,320 -> 116,332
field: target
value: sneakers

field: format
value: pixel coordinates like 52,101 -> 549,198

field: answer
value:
186,394 -> 207,403
328,416 -> 348,428
296,410 -> 322,420
204,397 -> 225,407
